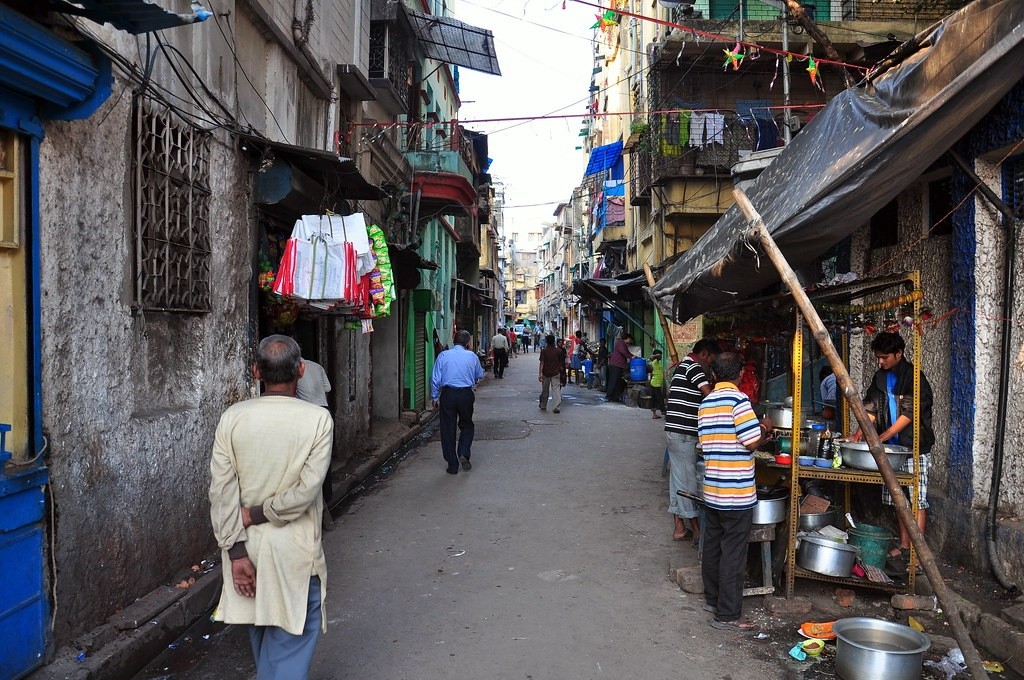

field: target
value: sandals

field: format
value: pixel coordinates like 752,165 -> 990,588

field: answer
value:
709,616 -> 755,631
906,562 -> 923,574
886,544 -> 910,560
702,603 -> 719,612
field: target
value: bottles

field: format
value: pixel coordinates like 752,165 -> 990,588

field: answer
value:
807,425 -> 825,458
818,423 -> 831,459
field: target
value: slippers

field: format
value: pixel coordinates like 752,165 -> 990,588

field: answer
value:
673,528 -> 693,540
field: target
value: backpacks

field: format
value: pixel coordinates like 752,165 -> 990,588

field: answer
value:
579,342 -> 587,360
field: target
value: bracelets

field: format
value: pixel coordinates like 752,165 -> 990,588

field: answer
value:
761,423 -> 769,433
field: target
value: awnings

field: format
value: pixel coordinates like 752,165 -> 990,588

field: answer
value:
572,272 -> 654,305
248,137 -> 395,200
480,267 -> 496,278
659,0 -> 1024,323
411,10 -> 502,78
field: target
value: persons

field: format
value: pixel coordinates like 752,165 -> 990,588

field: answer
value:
556,330 -> 596,390
597,339 -> 607,391
516,322 -> 544,354
650,349 -> 667,419
431,329 -> 483,474
820,365 -> 842,418
208,334 -> 335,680
299,354 -> 333,503
490,325 -> 517,379
664,338 -> 721,551
697,351 -> 774,630
839,332 -> 935,576
605,333 -> 635,402
538,334 -> 563,413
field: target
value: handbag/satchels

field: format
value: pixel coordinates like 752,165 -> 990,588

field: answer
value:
274,210 -> 374,314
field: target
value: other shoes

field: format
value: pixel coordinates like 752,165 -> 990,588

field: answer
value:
446,468 -> 457,474
539,405 -> 546,410
499,376 -> 503,379
459,456 -> 471,470
494,375 -> 498,378
554,408 -> 560,413
652,416 -> 662,419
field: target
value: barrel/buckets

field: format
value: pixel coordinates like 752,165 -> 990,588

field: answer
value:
640,396 -> 652,409
629,356 -> 647,381
846,524 -> 892,571
584,360 -> 593,379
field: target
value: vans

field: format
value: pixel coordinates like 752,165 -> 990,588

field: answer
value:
514,324 -> 532,345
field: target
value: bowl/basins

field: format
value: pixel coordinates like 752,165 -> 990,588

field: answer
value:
840,442 -> 913,471
799,458 -> 815,466
801,638 -> 824,656
815,459 -> 833,468
799,509 -> 837,531
775,455 -> 792,464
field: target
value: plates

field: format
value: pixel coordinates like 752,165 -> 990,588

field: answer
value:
796,627 -> 834,640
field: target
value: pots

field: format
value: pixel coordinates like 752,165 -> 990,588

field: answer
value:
755,474 -> 789,499
752,495 -> 790,524
796,535 -> 858,577
766,405 -> 807,429
832,617 -> 931,680
